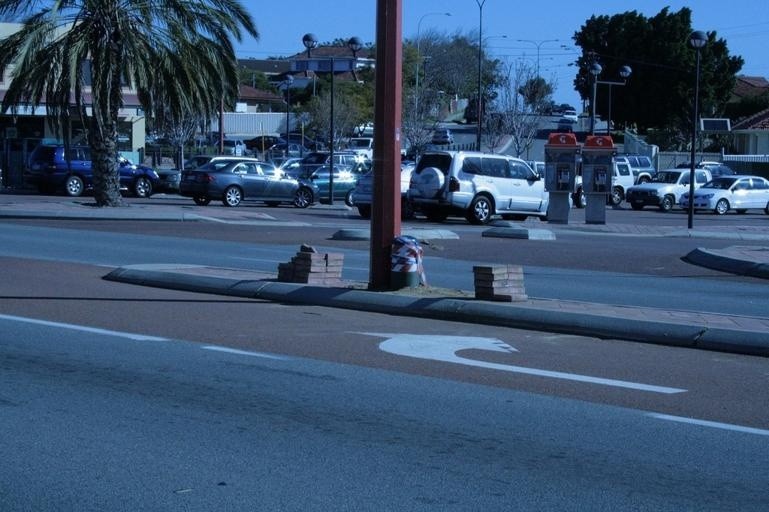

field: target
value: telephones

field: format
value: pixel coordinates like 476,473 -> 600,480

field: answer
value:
594,166 -> 607,192
557,167 -> 569,191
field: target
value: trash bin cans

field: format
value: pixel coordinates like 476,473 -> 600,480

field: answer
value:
391,235 -> 419,288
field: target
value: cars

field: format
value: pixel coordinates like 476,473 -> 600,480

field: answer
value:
562,109 -> 578,124
558,118 -> 573,133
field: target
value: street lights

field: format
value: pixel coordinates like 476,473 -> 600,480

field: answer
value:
687,29 -> 709,227
415,12 -> 452,118
589,60 -> 632,135
297,32 -> 364,205
481,34 -> 574,92
